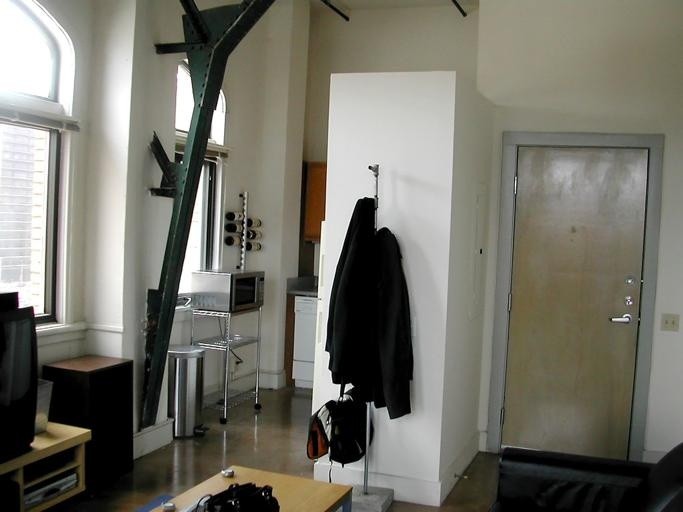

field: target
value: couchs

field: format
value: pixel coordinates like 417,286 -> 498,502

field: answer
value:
489,439 -> 681,511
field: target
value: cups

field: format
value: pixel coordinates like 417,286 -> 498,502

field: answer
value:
224,211 -> 262,252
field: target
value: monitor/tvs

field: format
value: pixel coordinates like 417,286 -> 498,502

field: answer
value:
0,307 -> 38,464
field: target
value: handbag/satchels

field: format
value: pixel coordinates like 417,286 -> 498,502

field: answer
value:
305,387 -> 373,463
204,482 -> 279,512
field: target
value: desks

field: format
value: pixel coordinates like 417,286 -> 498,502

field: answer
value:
148,464 -> 354,512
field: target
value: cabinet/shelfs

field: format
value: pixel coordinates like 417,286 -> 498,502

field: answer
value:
292,295 -> 317,389
0,418 -> 94,512
190,309 -> 262,425
42,352 -> 137,496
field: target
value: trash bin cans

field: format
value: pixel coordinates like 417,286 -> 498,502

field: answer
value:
168,344 -> 210,438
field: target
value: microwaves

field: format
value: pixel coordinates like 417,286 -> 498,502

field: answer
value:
189,271 -> 264,312
